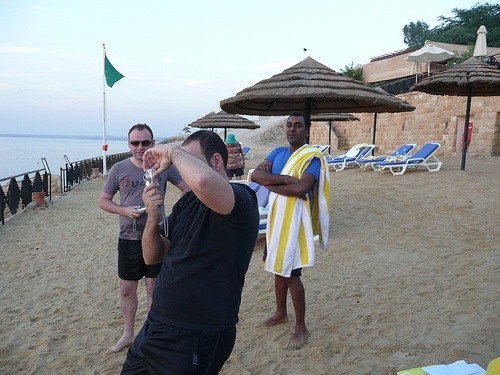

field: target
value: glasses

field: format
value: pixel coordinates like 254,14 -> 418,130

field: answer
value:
129,140 -> 152,147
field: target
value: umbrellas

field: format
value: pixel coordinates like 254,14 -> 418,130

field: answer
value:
188,109 -> 218,132
351,86 -> 416,156
197,109 -> 260,141
409,56 -> 500,171
310,113 -> 360,154
220,57 -> 416,145
404,43 -> 455,77
472,25 -> 488,61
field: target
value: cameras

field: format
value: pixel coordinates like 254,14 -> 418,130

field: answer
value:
137,207 -> 147,213
143,169 -> 158,186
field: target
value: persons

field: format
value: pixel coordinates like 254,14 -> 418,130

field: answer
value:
251,112 -> 322,350
223,133 -> 247,180
120,130 -> 260,375
98,124 -> 192,355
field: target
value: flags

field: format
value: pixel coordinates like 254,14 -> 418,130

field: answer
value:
104,53 -> 124,88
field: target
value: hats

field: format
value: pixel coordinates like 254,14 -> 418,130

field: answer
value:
224,134 -> 237,144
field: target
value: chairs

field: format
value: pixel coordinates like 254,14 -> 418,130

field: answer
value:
242,146 -> 254,159
370,143 -> 444,176
355,144 -> 416,172
311,143 -> 375,172
